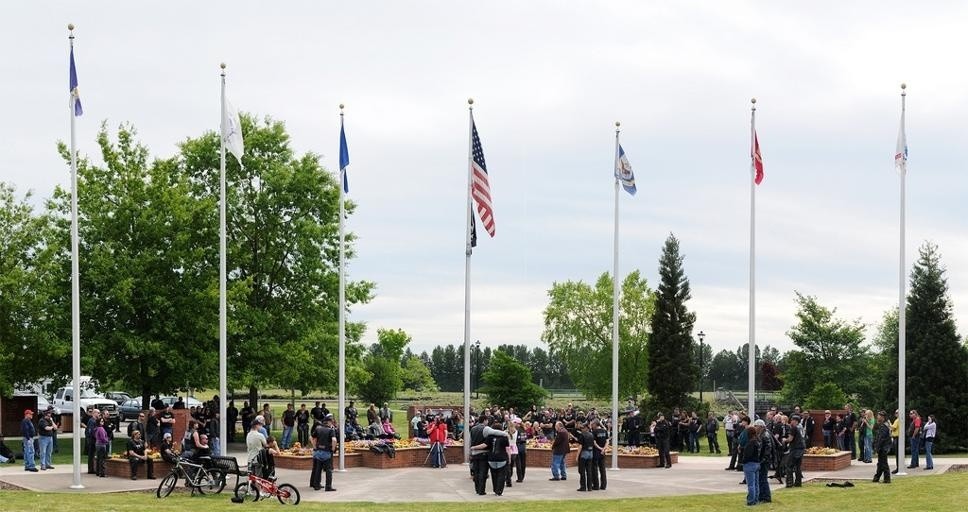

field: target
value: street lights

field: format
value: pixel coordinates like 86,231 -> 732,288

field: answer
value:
473,338 -> 482,397
698,328 -> 708,403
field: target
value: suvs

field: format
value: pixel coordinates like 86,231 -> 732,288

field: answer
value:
16,392 -> 63,429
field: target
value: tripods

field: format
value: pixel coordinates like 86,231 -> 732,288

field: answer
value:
424,426 -> 448,469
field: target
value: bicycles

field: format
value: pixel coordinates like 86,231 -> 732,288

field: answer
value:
157,446 -> 228,498
235,452 -> 301,506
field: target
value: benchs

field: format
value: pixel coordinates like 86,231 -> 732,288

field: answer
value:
186,454 -> 249,493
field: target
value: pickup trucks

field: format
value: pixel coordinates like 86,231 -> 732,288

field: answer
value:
101,390 -> 134,406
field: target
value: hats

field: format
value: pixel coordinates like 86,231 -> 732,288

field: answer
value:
753,419 -> 768,428
24,409 -> 35,415
254,414 -> 266,426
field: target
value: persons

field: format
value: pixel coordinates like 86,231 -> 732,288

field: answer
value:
469,405 -> 612,496
21,410 -> 39,472
226,401 -> 338,491
38,411 -> 57,470
822,403 -> 936,484
617,407 -> 720,469
411,407 -> 464,470
48,407 -> 61,453
344,400 -> 396,440
723,407 -> 815,504
86,395 -> 220,481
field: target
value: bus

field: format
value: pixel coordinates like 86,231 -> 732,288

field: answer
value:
14,374 -> 102,397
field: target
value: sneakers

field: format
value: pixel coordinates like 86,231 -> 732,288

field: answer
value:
705,449 -> 742,472
665,463 -> 672,469
739,475 -> 802,507
850,457 -> 933,483
472,475 -> 608,497
655,462 -> 665,468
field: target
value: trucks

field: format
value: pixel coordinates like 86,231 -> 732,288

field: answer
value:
20,378 -> 120,431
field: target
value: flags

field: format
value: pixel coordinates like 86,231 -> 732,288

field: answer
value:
70,46 -> 82,116
471,108 -> 495,247
339,114 -> 350,197
614,130 -> 636,196
221,80 -> 245,171
895,92 -> 910,173
751,111 -> 763,185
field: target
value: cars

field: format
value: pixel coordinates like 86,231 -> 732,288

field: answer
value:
159,397 -> 205,411
115,397 -> 155,421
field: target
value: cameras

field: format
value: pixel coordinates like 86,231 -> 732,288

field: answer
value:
435,419 -> 440,426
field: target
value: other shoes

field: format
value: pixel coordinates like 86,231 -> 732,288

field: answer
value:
46,466 -> 54,469
88,471 -> 95,474
132,475 -> 136,480
100,474 -> 107,477
315,486 -> 320,489
147,475 -> 157,479
28,468 -> 38,472
41,465 -> 46,470
324,488 -> 336,491
25,469 -> 29,471
